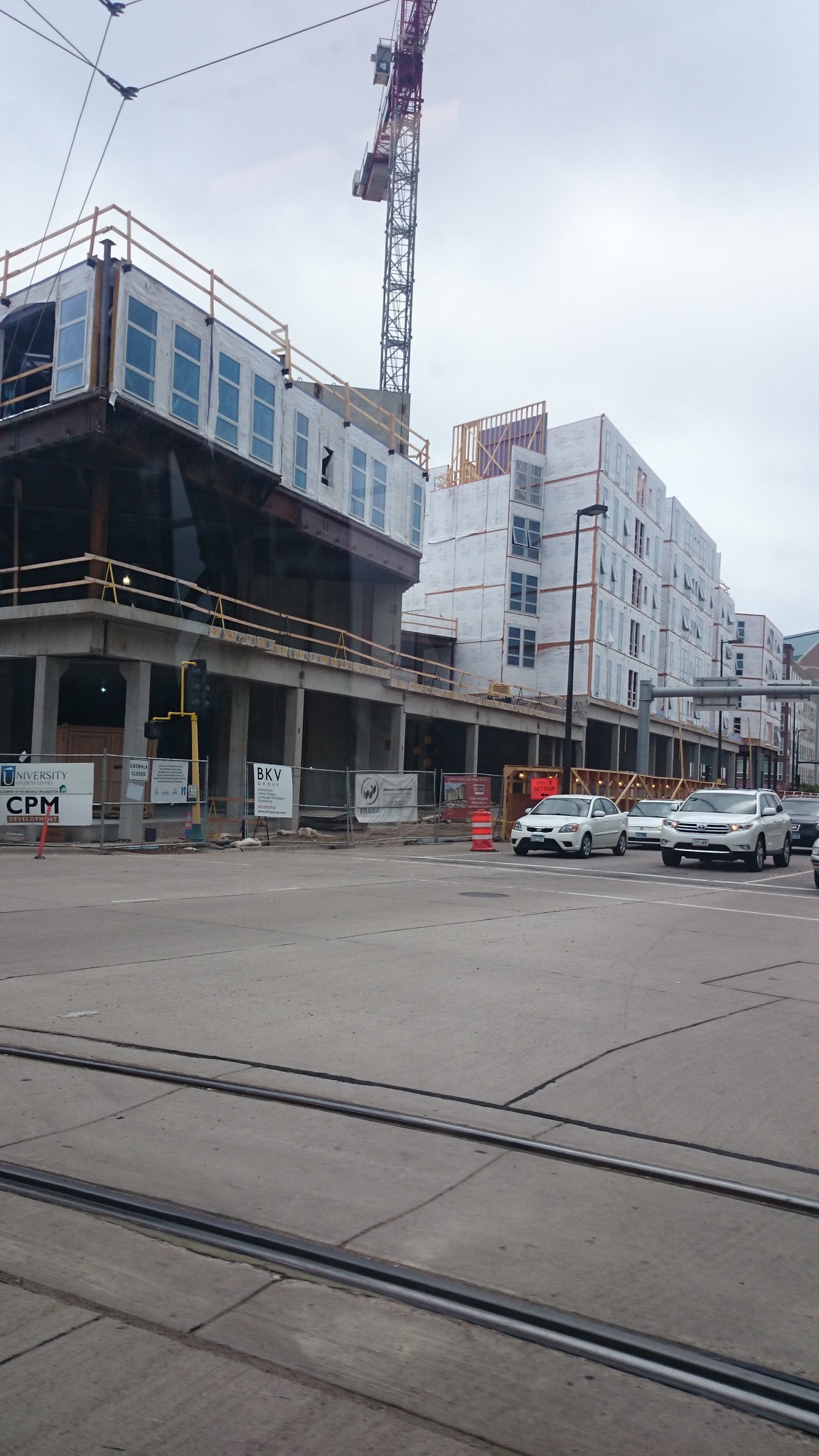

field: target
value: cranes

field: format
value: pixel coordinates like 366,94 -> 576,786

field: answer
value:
343,0 -> 437,398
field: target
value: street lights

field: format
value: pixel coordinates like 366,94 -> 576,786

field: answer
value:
560,504 -> 608,794
794,729 -> 807,790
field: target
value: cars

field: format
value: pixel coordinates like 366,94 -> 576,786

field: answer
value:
659,786 -> 792,872
811,838 -> 819,888
623,798 -> 686,846
780,795 -> 819,847
511,794 -> 628,858
